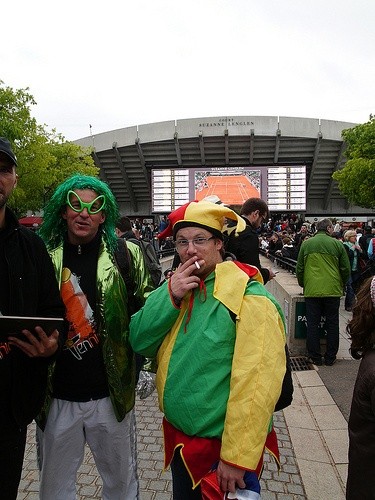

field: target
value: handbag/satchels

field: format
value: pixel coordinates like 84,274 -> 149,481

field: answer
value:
358,256 -> 367,269
344,285 -> 356,312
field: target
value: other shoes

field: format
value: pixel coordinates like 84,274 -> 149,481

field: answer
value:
306,356 -> 322,366
326,357 -> 336,366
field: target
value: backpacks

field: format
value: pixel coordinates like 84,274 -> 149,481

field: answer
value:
128,239 -> 162,288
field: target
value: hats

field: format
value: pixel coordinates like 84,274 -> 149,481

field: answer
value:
0,137 -> 19,166
153,198 -> 247,243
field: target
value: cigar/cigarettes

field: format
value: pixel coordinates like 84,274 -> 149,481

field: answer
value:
194,260 -> 201,270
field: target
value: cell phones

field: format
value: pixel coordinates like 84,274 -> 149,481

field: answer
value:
274,271 -> 280,274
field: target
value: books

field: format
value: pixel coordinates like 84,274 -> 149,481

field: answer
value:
0,310 -> 64,343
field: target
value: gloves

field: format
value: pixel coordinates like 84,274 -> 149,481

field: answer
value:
135,370 -> 156,400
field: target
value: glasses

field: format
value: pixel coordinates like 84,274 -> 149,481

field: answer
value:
173,234 -> 214,247
66,191 -> 107,214
260,215 -> 267,223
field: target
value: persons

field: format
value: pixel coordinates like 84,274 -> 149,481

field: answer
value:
109,212 -> 176,258
34,174 -> 158,500
126,193 -> 293,500
295,217 -> 351,367
255,209 -> 375,312
346,275 -> 375,500
222,198 -> 278,286
113,214 -> 162,288
0,138 -> 67,500
